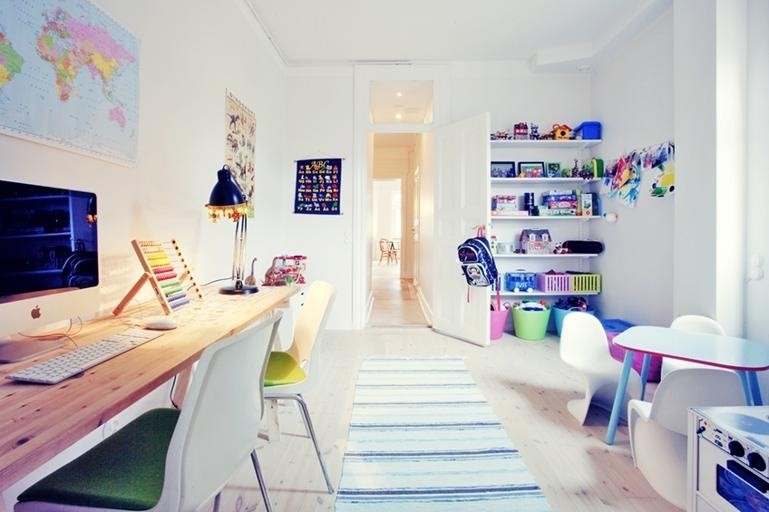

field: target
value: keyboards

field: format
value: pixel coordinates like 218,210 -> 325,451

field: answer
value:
6,326 -> 166,389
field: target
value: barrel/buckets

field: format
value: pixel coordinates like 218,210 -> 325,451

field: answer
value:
490,303 -> 511,340
511,301 -> 553,341
553,305 -> 597,337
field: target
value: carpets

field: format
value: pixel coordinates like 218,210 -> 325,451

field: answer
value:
335,355 -> 553,511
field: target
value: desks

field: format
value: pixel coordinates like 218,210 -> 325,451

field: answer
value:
0,283 -> 301,511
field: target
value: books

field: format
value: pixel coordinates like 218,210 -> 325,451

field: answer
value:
491,188 -> 598,217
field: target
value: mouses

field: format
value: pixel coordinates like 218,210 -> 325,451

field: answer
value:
146,317 -> 179,330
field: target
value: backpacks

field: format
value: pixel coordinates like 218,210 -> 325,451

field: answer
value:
457,228 -> 501,311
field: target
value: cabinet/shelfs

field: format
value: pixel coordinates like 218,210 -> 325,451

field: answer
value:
489,140 -> 605,297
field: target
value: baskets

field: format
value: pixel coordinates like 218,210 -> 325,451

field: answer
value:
536,271 -> 572,293
572,274 -> 601,293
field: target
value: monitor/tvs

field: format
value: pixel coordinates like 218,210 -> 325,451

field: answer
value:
0,180 -> 102,363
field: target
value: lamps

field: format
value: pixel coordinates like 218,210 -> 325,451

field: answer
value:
204,164 -> 259,295
